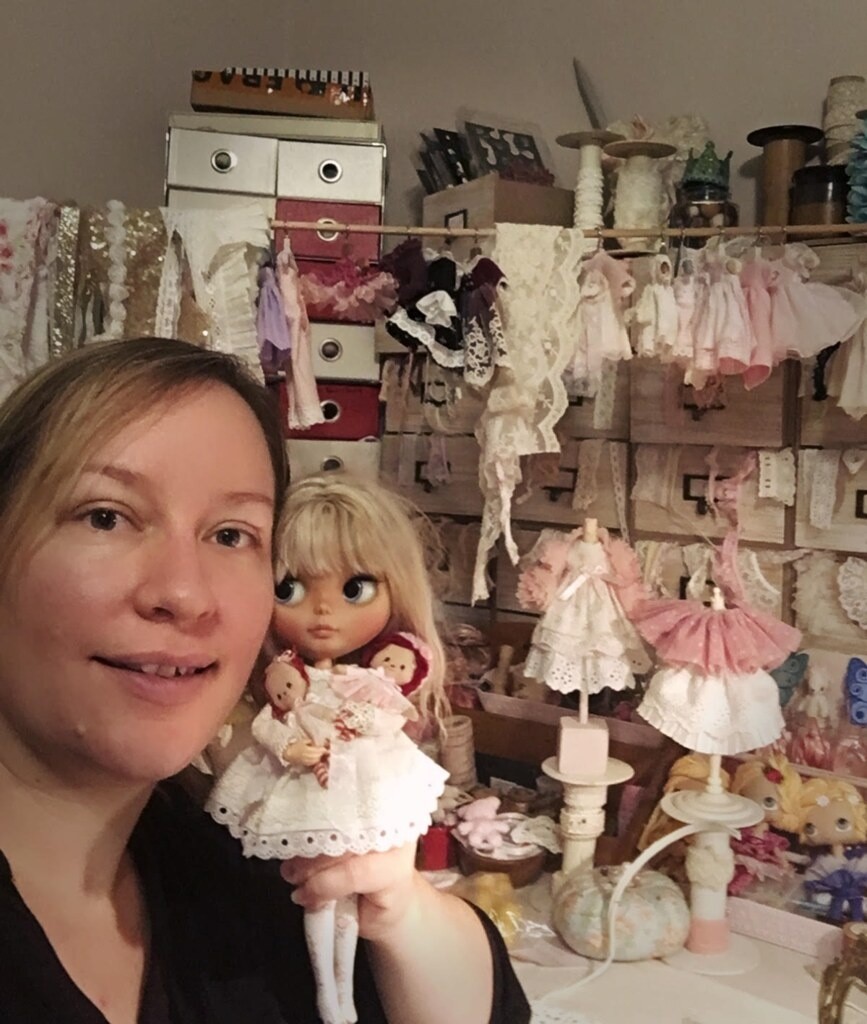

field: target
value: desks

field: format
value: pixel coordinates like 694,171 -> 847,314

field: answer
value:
511,931 -> 830,1024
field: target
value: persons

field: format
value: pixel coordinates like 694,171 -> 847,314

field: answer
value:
208,473 -> 451,1024
0,333 -> 538,1024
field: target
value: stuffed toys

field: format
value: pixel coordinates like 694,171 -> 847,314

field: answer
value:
333,630 -> 429,741
662,755 -> 867,930
259,650 -> 346,792
455,795 -> 510,849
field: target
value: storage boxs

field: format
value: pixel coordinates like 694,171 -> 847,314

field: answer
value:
162,126 -> 867,966
